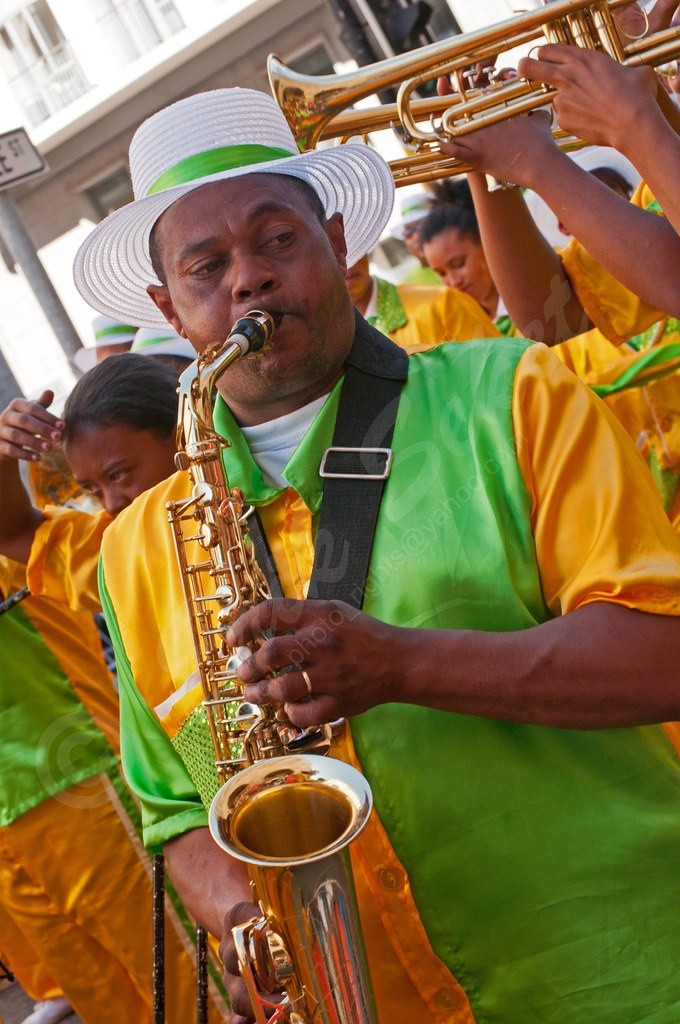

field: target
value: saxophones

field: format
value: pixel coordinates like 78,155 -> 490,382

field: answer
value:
164,305 -> 372,1024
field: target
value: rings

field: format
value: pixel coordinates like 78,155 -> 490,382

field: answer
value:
301,670 -> 312,694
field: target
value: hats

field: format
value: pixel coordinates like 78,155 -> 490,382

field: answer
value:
130,326 -> 198,358
71,88 -> 396,330
391,193 -> 429,241
73,315 -> 137,373
521,145 -> 643,250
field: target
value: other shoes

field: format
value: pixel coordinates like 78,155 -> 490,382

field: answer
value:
19,997 -> 72,1024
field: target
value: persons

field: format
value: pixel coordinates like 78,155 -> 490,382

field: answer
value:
341,0 -> 680,551
0,319 -> 207,1024
73,89 -> 679,1024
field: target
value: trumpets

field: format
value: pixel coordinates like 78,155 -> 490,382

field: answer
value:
262,1 -> 680,155
289,58 -> 680,187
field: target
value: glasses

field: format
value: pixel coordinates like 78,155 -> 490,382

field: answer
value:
402,222 -> 422,237
